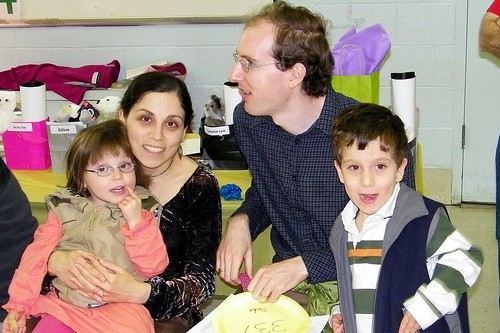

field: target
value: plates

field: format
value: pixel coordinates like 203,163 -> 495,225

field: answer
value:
211,291 -> 312,333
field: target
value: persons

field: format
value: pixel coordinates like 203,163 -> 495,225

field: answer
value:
330,103 -> 484,333
1,119 -> 170,333
0,157 -> 53,333
214,0 -> 416,333
47,71 -> 222,333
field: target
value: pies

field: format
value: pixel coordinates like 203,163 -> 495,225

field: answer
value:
213,290 -> 312,333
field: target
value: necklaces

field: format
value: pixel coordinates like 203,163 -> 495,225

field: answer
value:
150,155 -> 174,177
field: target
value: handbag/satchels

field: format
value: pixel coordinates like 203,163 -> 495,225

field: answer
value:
330,44 -> 380,105
45,119 -> 87,173
2,117 -> 51,171
199,117 -> 248,170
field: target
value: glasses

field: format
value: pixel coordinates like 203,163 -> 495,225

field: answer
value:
233,52 -> 283,73
83,160 -> 136,177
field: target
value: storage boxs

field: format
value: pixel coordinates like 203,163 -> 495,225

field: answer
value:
45,118 -> 97,175
1,117 -> 50,170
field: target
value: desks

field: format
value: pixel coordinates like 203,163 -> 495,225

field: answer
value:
11,143 -> 422,296
